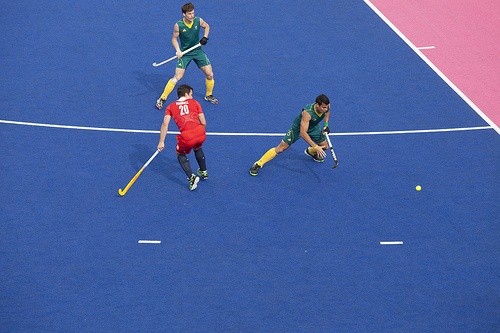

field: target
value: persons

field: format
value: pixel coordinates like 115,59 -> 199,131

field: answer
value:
155,3 -> 219,109
249,94 -> 330,177
157,84 -> 208,190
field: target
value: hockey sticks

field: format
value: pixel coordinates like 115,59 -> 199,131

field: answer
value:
324,133 -> 339,169
118,150 -> 160,196
152,44 -> 202,67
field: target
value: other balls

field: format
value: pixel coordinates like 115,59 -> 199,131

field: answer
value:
415,185 -> 422,191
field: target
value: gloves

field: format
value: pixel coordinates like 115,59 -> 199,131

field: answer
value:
199,37 -> 208,46
322,126 -> 330,136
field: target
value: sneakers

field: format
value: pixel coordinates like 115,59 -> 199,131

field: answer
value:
204,95 -> 218,104
156,97 -> 166,109
187,174 -> 199,191
304,147 -> 324,162
250,162 -> 261,176
197,168 -> 208,179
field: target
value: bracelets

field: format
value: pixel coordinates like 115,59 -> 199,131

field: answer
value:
314,145 -> 318,148
323,122 -> 328,126
